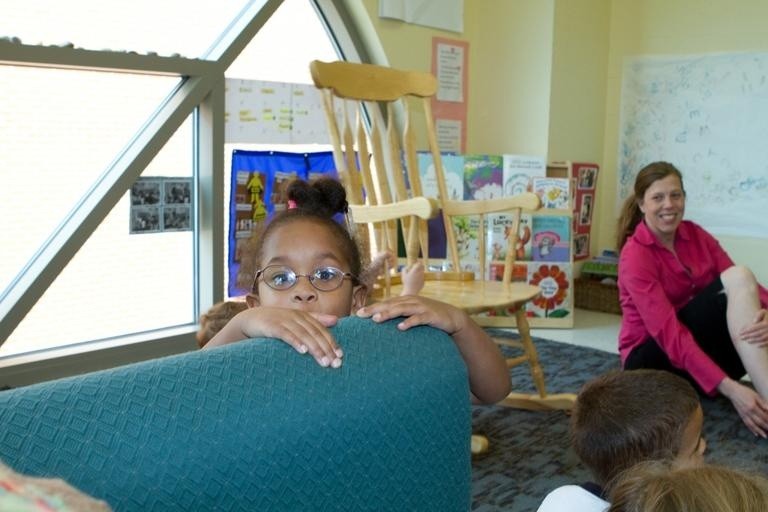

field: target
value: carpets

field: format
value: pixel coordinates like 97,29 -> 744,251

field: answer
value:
470,327 -> 768,512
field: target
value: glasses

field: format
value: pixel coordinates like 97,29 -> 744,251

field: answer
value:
250,263 -> 362,292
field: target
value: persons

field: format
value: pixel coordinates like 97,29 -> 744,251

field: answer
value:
192,175 -> 513,406
598,451 -> 768,512
613,158 -> 768,440
196,250 -> 428,354
536,369 -> 707,512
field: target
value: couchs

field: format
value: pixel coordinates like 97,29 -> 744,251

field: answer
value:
0,311 -> 475,512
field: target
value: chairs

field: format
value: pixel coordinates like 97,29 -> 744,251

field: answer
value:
309,58 -> 580,456
